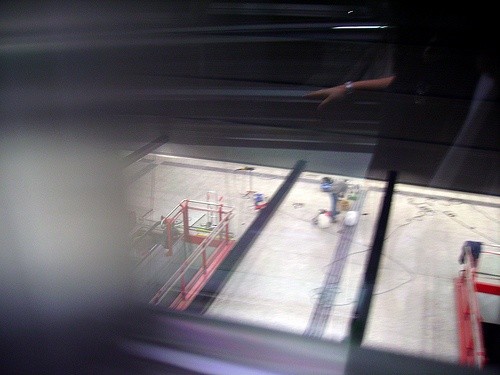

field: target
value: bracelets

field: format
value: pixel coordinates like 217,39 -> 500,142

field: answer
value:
344,80 -> 353,99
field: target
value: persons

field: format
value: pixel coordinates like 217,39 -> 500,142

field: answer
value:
305,44 -> 434,178
